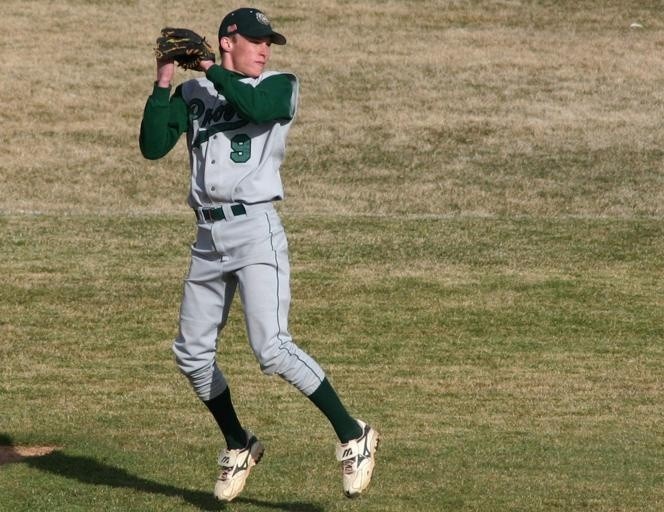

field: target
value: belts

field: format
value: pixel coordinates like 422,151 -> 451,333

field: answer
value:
193,205 -> 246,223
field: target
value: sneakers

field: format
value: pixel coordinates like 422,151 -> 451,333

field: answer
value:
335,418 -> 380,499
213,429 -> 265,505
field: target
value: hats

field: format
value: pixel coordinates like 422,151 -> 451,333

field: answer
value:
218,8 -> 286,45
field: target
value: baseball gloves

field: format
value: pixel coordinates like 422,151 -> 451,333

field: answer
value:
154,27 -> 215,71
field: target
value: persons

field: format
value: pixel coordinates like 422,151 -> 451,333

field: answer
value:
139,8 -> 382,502
